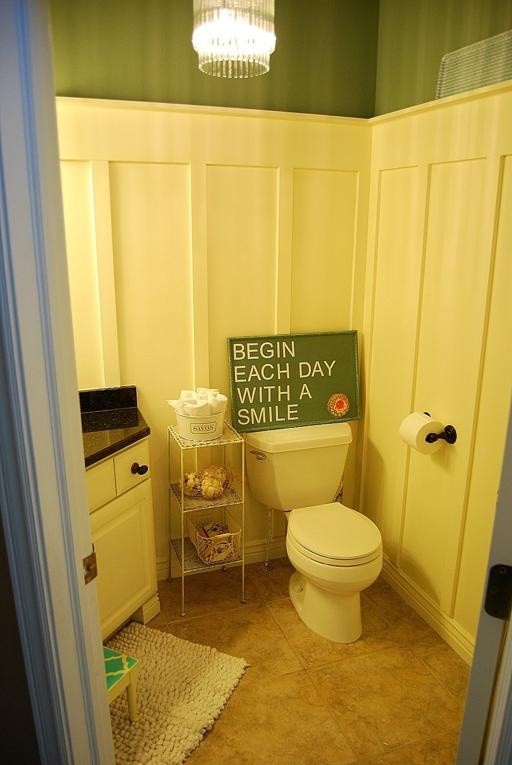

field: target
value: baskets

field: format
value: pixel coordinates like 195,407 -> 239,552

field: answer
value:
187,512 -> 241,563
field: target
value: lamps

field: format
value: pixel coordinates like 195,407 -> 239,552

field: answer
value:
192,0 -> 277,82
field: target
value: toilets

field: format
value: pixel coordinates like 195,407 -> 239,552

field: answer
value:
244,423 -> 384,645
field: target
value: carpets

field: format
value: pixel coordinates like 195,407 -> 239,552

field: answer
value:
99,619 -> 249,764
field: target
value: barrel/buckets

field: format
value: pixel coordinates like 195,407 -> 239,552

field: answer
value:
175,409 -> 225,441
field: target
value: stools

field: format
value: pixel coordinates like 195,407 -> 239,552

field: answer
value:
98,644 -> 140,726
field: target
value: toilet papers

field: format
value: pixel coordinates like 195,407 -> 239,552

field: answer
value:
398,413 -> 444,455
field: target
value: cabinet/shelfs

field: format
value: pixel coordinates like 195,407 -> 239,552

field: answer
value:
84,430 -> 164,642
164,424 -> 250,617
